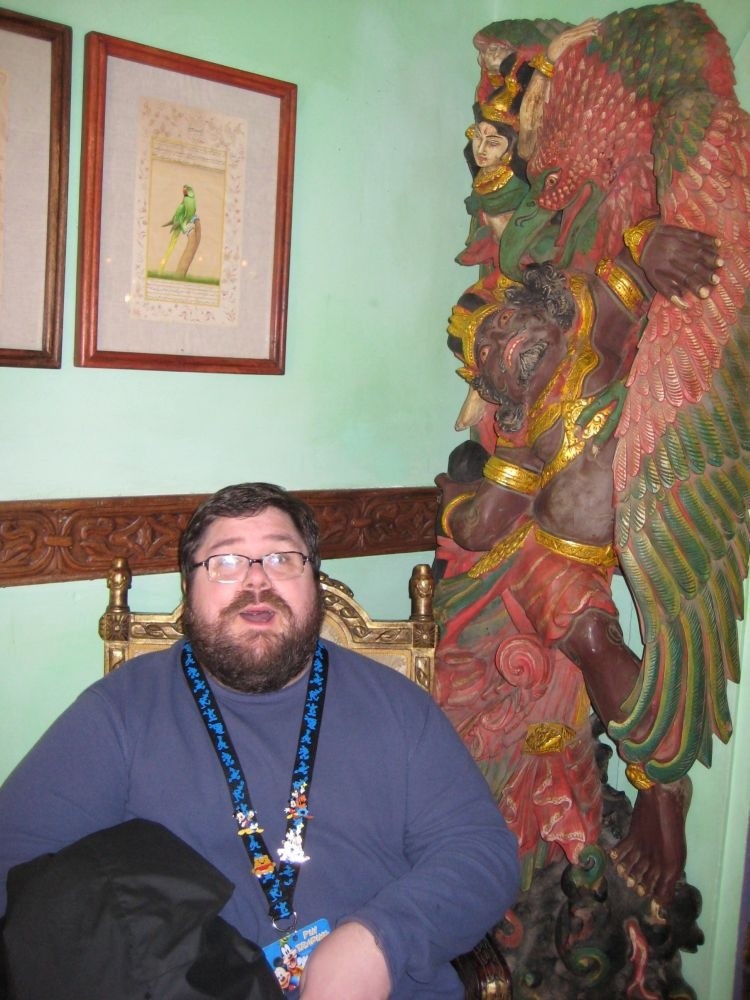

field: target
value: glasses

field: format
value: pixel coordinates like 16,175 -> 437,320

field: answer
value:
194,552 -> 308,585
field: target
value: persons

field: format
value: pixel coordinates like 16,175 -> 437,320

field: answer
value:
0,481 -> 522,1000
434,54 -> 727,904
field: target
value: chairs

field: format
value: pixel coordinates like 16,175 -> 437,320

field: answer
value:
99,553 -> 436,702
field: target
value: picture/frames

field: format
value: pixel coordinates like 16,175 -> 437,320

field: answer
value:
73,31 -> 297,379
0,6 -> 77,370
